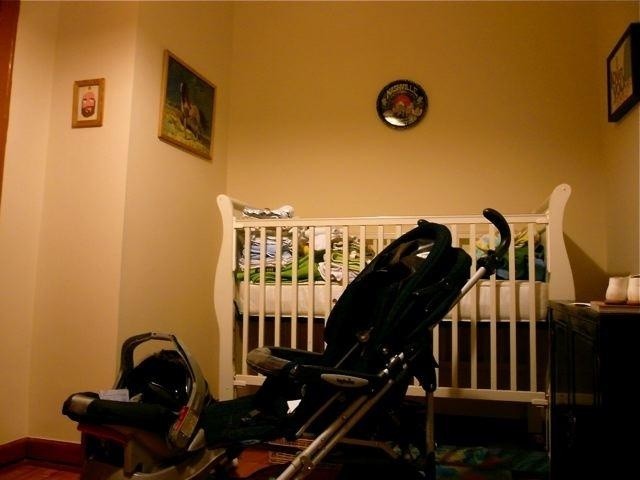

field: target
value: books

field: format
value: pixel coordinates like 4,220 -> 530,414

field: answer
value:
589,298 -> 640,314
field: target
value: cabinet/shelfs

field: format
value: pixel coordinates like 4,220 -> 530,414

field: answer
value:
546,299 -> 640,479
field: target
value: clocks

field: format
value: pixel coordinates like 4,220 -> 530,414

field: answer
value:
376,79 -> 428,129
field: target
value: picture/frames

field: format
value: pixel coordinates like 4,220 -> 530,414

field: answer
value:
72,78 -> 103,129
158,49 -> 217,162
606,21 -> 639,122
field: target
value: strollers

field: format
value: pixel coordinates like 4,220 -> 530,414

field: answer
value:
62,208 -> 515,479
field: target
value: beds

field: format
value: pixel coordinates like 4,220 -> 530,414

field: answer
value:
211,184 -> 573,451
